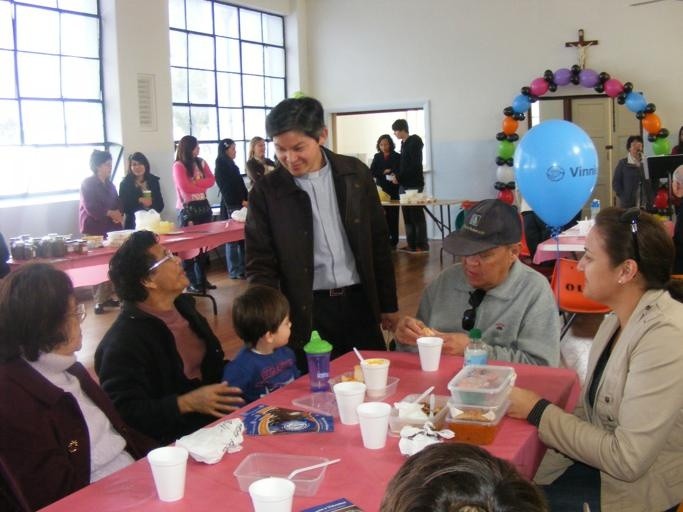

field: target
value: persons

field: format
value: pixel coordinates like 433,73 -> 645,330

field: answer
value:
1,97 -> 682,512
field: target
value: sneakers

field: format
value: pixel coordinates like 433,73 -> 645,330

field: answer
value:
399,247 -> 429,254
229,274 -> 244,279
203,281 -> 217,289
187,281 -> 204,293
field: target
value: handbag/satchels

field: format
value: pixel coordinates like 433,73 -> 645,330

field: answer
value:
226,205 -> 240,219
182,199 -> 212,221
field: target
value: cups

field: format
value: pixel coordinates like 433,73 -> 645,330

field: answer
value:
147,447 -> 188,503
415,336 -> 443,372
333,381 -> 366,424
143,191 -> 152,198
463,330 -> 489,368
248,478 -> 296,512
358,401 -> 391,449
361,357 -> 391,396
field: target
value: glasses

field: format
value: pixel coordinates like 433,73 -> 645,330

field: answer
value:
461,288 -> 486,331
620,207 -> 640,267
66,304 -> 85,321
147,249 -> 174,271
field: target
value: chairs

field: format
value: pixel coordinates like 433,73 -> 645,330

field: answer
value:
463,202 -> 531,265
551,257 -> 616,345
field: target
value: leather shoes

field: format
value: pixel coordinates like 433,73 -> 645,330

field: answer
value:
94,299 -> 119,314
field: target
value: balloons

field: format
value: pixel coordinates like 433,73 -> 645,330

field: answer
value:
494,64 -> 671,238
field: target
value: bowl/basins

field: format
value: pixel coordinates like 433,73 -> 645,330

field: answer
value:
232,452 -> 328,497
390,393 -> 449,434
328,371 -> 400,400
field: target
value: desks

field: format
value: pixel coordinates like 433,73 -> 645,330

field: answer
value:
380,198 -> 468,270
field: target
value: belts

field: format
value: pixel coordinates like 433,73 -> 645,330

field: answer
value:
313,284 -> 362,296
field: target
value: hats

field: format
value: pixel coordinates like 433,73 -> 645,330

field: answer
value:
443,199 -> 522,256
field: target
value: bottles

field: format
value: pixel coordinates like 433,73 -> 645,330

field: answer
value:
9,233 -> 88,259
304,331 -> 333,393
590,198 -> 600,220
